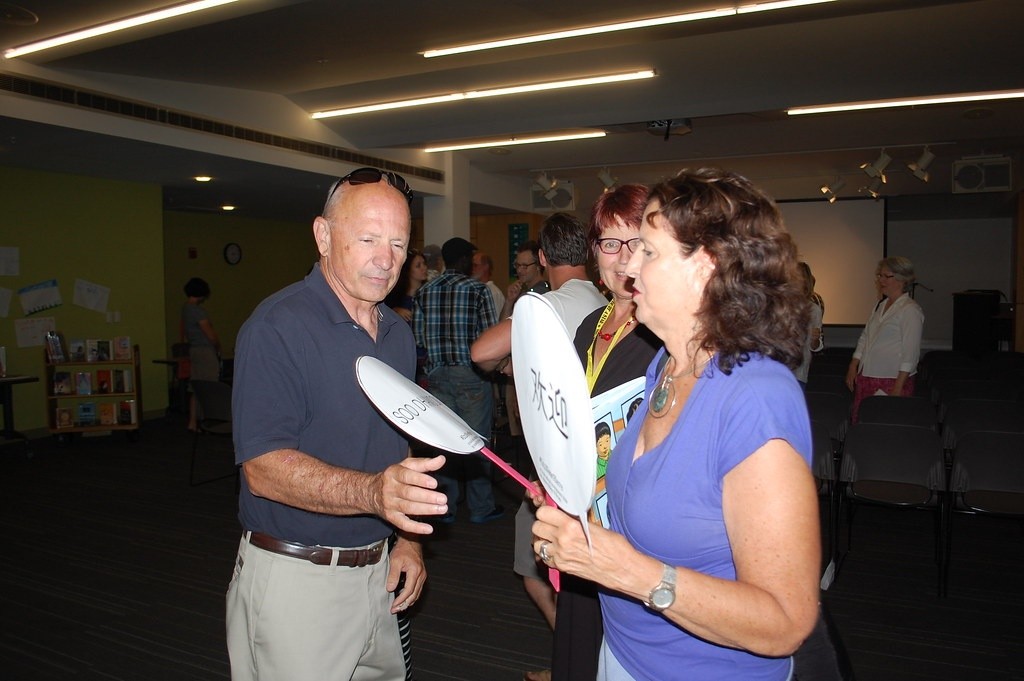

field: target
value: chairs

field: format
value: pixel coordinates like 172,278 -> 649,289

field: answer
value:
189,380 -> 239,488
794,346 -> 1024,597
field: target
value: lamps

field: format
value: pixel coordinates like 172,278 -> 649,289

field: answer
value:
3,0 -> 1024,204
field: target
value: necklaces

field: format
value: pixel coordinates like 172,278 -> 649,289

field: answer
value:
649,352 -> 713,419
598,315 -> 636,341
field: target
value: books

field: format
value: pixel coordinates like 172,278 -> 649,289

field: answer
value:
45,332 -> 135,429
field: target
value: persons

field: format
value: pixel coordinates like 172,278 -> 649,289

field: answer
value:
527,185 -> 670,681
531,166 -> 822,681
470,211 -> 609,681
384,238 -> 551,522
792,262 -> 824,390
226,168 -> 448,681
181,277 -> 221,433
846,257 -> 924,426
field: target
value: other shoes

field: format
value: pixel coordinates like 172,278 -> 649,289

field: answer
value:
433,510 -> 455,523
470,503 -> 506,522
186,426 -> 206,436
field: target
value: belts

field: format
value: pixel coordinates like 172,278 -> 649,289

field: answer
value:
243,530 -> 386,568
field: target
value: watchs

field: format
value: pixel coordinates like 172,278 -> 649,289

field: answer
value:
644,563 -> 676,612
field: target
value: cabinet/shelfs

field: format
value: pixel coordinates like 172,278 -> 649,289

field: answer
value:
43,343 -> 143,444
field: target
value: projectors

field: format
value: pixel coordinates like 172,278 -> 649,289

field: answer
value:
646,117 -> 692,135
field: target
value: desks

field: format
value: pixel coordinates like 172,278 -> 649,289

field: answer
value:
0,376 -> 38,446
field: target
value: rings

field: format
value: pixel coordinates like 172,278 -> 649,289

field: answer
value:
540,540 -> 550,560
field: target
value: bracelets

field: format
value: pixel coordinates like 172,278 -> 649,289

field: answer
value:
495,357 -> 509,373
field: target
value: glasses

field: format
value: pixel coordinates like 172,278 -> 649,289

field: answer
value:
596,234 -> 641,255
876,272 -> 896,280
323,167 -> 414,218
406,247 -> 420,255
512,257 -> 537,270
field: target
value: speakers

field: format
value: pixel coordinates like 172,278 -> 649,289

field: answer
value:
951,157 -> 1012,192
532,183 -> 576,210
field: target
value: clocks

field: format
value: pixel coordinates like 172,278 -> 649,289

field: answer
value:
224,243 -> 242,265
